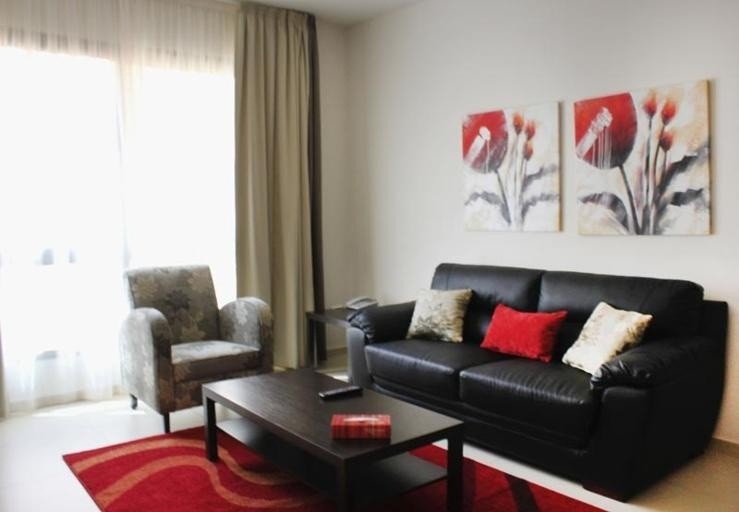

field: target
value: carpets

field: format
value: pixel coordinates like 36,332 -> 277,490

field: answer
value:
62,424 -> 607,512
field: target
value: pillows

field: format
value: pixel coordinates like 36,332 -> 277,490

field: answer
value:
478,299 -> 653,380
403,287 -> 473,346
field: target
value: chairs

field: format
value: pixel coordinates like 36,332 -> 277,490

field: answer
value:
121,263 -> 275,439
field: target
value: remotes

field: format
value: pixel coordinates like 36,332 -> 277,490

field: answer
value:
317,384 -> 363,400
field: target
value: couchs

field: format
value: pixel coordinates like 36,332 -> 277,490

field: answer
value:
346,261 -> 731,503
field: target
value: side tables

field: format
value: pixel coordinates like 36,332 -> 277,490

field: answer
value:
305,305 -> 360,371
